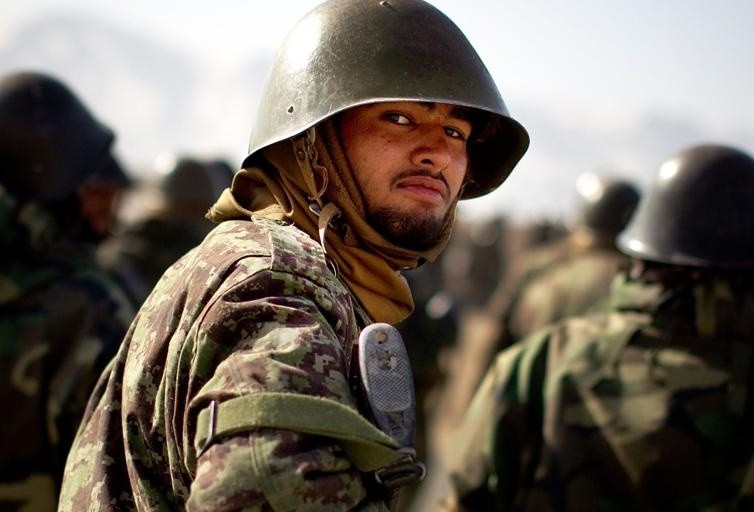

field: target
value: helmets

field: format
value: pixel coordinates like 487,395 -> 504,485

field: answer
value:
147,152 -> 214,204
239,1 -> 531,201
2,71 -> 115,192
78,153 -> 137,192
611,139 -> 754,271
562,168 -> 647,237
209,156 -> 238,206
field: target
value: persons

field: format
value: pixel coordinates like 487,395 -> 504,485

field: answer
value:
1,70 -> 237,512
410,145 -> 753,512
55,2 -> 531,512
